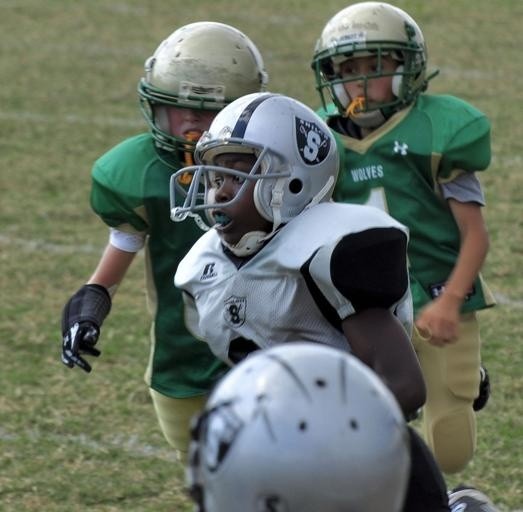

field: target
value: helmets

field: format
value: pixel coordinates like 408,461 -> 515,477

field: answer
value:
137,20 -> 268,172
170,92 -> 340,258
311,2 -> 429,129
181,341 -> 413,511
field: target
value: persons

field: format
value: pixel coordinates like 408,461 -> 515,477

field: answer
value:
169,90 -> 500,512
60,20 -> 274,470
306,2 -> 497,474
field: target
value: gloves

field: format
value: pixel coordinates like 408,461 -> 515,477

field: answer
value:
60,283 -> 111,372
